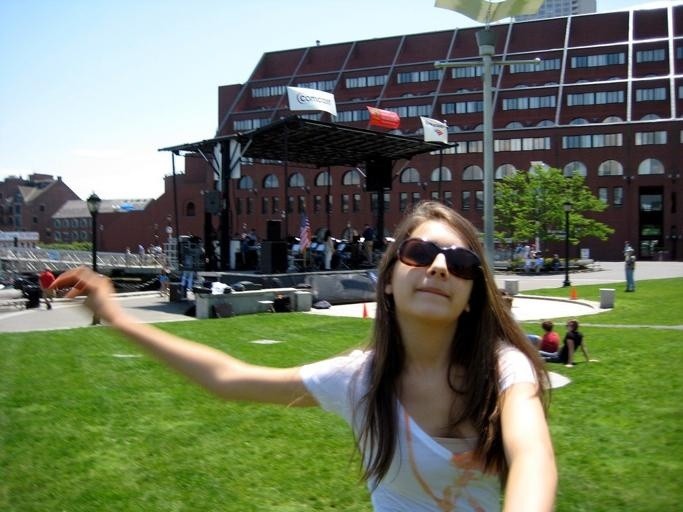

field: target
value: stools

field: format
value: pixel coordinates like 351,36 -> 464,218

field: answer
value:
505,279 -> 519,295
258,300 -> 275,311
599,288 -> 616,308
294,292 -> 313,311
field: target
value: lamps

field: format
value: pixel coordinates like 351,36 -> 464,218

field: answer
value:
249,189 -> 258,196
418,182 -> 429,191
668,173 -> 681,183
302,186 -> 310,194
623,176 -> 635,184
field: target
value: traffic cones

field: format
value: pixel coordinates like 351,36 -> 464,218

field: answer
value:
570,286 -> 578,300
363,304 -> 369,319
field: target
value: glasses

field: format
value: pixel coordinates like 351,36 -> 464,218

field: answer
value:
394,235 -> 482,281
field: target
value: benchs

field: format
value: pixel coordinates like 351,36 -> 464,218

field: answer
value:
194,288 -> 296,318
512,261 -> 580,274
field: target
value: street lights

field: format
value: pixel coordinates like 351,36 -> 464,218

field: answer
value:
346,221 -> 353,242
242,221 -> 247,233
563,199 -> 573,287
280,209 -> 286,240
87,190 -> 101,324
166,213 -> 173,267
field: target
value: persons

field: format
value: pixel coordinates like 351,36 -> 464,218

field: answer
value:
537,319 -> 601,369
298,215 -> 395,271
521,320 -> 559,352
185,223 -> 260,270
510,242 -> 561,276
624,247 -> 636,292
623,240 -> 630,257
39,266 -> 55,309
125,242 -> 165,266
45,198 -> 559,511
158,267 -> 168,297
